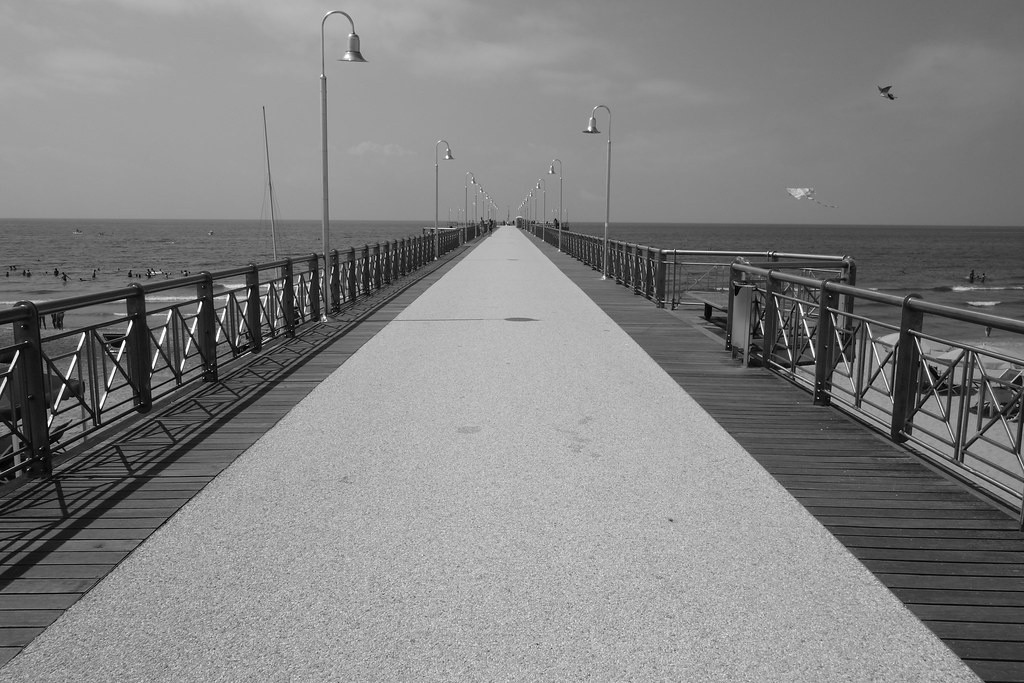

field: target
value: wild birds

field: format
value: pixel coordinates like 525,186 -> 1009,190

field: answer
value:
876,84 -> 898,100
5,264 -> 192,282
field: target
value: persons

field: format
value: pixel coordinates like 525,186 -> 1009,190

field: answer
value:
51,311 -> 64,329
488,217 -> 492,234
981,272 -> 986,282
92,269 -> 96,277
128,269 -> 133,277
184,269 -> 188,275
54,268 -> 70,281
6,265 -> 16,277
478,217 -> 484,237
970,269 -> 975,278
76,228 -> 82,233
38,314 -> 47,329
146,268 -> 171,277
23,269 -> 30,276
513,220 -> 514,224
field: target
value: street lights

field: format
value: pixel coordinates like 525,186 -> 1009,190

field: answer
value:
434,139 -> 454,261
535,178 -> 546,244
473,182 -> 483,238
318,9 -> 367,321
481,189 -> 537,237
582,103 -> 613,280
548,159 -> 564,253
464,172 -> 474,242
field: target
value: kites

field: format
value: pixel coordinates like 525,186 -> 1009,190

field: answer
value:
785,186 -> 839,209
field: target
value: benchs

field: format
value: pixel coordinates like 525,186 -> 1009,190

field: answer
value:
684,290 -> 767,322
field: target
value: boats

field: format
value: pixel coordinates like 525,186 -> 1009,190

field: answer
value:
72,229 -> 84,235
102,333 -> 127,354
208,230 -> 214,236
227,107 -> 305,289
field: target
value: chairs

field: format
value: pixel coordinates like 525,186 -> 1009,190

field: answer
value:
0,432 -> 26,470
102,333 -> 129,355
49,418 -> 73,455
